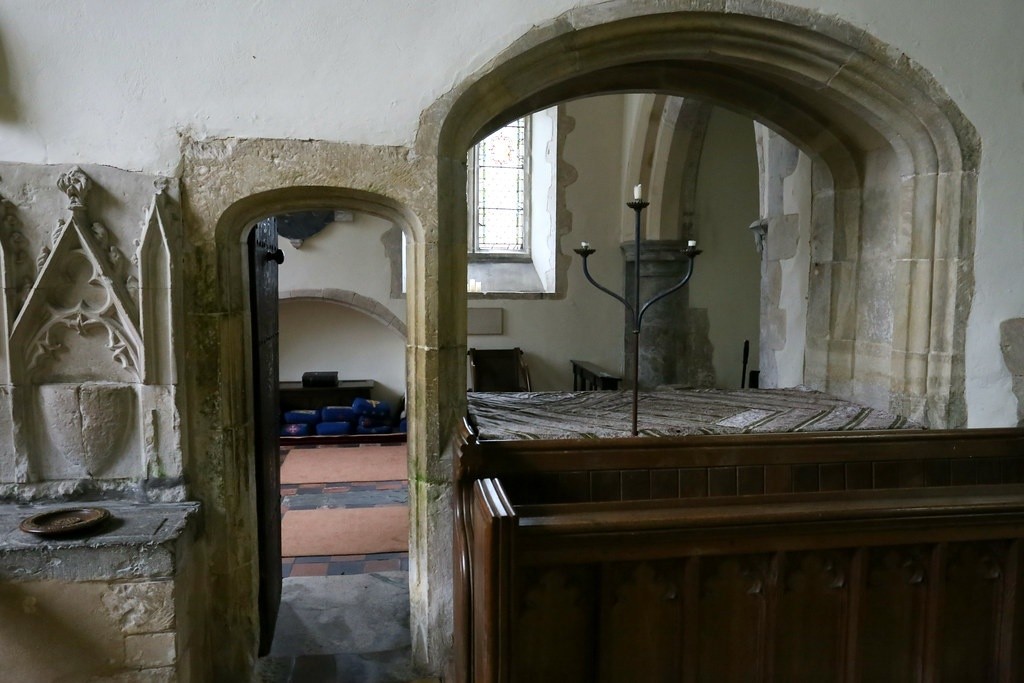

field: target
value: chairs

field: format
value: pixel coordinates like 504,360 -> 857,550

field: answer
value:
466,348 -> 532,392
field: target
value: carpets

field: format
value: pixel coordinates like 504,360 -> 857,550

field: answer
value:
280,446 -> 407,484
281,505 -> 410,557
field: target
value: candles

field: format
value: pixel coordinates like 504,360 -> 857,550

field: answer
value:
581,241 -> 589,246
688,240 -> 696,246
634,184 -> 642,199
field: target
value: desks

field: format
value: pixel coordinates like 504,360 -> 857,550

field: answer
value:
569,359 -> 623,391
277,379 -> 374,425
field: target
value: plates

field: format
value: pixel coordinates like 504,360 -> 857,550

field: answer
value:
20,506 -> 111,540
